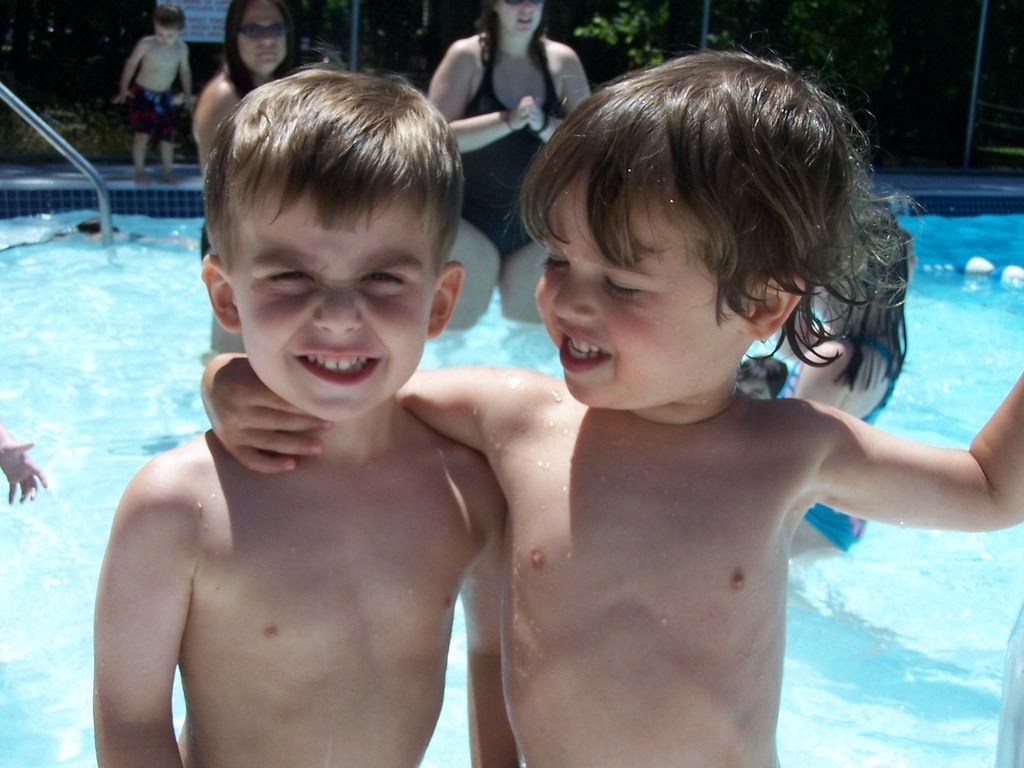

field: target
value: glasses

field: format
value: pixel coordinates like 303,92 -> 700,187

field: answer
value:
504,0 -> 543,6
237,21 -> 289,38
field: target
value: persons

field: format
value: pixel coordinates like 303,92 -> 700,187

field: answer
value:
199,52 -> 1024,768
725,218 -> 911,564
736,355 -> 789,398
113,5 -> 193,184
424,0 -> 591,330
91,66 -> 521,768
188,0 -> 297,357
